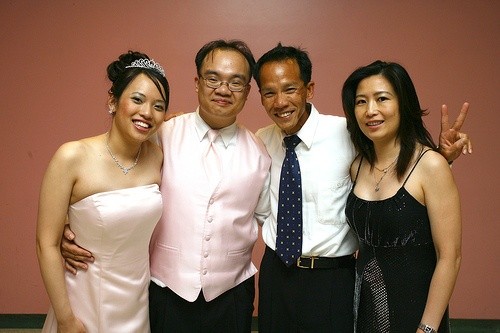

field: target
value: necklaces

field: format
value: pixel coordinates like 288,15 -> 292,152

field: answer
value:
102,133 -> 143,174
367,152 -> 399,192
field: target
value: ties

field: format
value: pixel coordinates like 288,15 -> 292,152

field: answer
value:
275,134 -> 302,267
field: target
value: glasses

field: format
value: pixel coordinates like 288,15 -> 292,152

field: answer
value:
199,72 -> 249,91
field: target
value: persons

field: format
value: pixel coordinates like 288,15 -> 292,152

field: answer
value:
340,59 -> 463,333
34,49 -> 170,333
60,39 -> 271,333
249,41 -> 473,333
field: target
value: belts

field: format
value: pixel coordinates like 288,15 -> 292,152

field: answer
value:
264,245 -> 356,269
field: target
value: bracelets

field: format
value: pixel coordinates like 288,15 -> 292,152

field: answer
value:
418,323 -> 436,333
